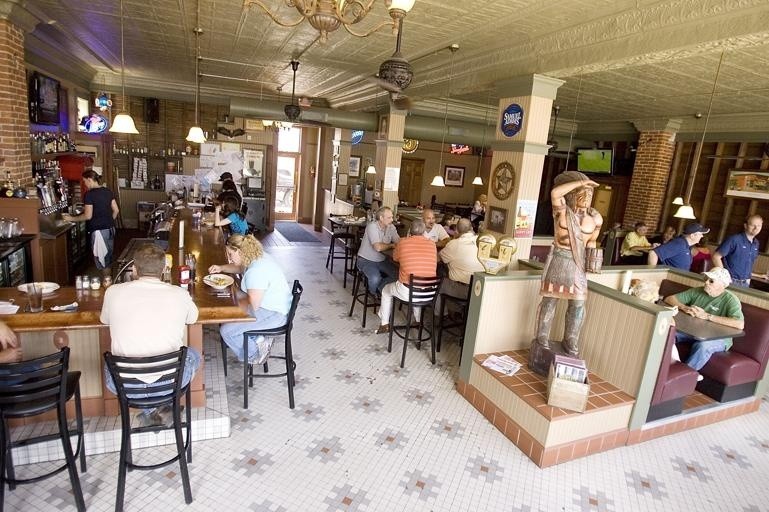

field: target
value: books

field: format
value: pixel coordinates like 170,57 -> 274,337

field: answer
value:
552,354 -> 590,382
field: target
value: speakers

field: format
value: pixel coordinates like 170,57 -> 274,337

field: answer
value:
143,98 -> 159,123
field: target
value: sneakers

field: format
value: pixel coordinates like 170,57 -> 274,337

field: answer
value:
412,320 -> 421,327
137,404 -> 184,433
247,335 -> 274,366
377,322 -> 390,335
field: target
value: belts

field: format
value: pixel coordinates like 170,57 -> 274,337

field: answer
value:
733,278 -> 746,284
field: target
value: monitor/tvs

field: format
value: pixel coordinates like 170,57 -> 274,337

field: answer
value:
575,147 -> 614,176
29,70 -> 60,125
246,176 -> 262,192
470,213 -> 484,224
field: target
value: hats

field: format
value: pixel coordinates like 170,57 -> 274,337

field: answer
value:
665,226 -> 676,235
701,267 -> 731,287
219,173 -> 232,181
684,222 -> 710,234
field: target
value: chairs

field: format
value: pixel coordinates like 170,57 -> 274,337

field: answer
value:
324,212 -> 475,369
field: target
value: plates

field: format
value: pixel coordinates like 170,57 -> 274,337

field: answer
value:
340,216 -> 367,224
188,202 -> 205,207
17,281 -> 60,293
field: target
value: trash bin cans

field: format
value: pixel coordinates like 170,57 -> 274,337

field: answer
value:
138,201 -> 156,233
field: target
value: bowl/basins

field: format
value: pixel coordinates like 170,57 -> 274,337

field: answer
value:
203,273 -> 234,289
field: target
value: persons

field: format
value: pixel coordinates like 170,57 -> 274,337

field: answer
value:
63,171 -> 120,268
647,221 -> 710,273
469,194 -> 487,221
216,171 -> 249,233
101,242 -> 201,433
619,221 -> 660,266
431,218 -> 488,321
406,208 -> 451,248
356,206 -> 403,300
536,170 -> 604,353
207,235 -> 291,365
710,214 -> 764,288
0,320 -> 25,366
375,219 -> 439,335
648,226 -> 678,245
663,267 -> 745,371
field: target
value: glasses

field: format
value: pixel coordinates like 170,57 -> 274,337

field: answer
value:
224,240 -> 234,246
704,277 -> 714,283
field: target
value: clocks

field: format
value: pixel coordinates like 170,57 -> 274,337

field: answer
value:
485,205 -> 509,234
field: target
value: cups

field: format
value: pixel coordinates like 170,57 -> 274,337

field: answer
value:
27,283 -> 44,312
586,246 -> 605,275
61,213 -> 69,223
0,215 -> 24,238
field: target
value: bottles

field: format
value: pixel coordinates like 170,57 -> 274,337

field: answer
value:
112,140 -> 146,154
34,158 -> 61,179
153,171 -> 161,189
75,275 -> 82,289
82,275 -> 90,289
149,144 -> 180,156
193,143 -> 198,155
91,276 -> 101,289
186,254 -> 196,280
193,213 -> 199,224
3,170 -> 14,190
103,276 -> 112,290
30,130 -> 71,152
